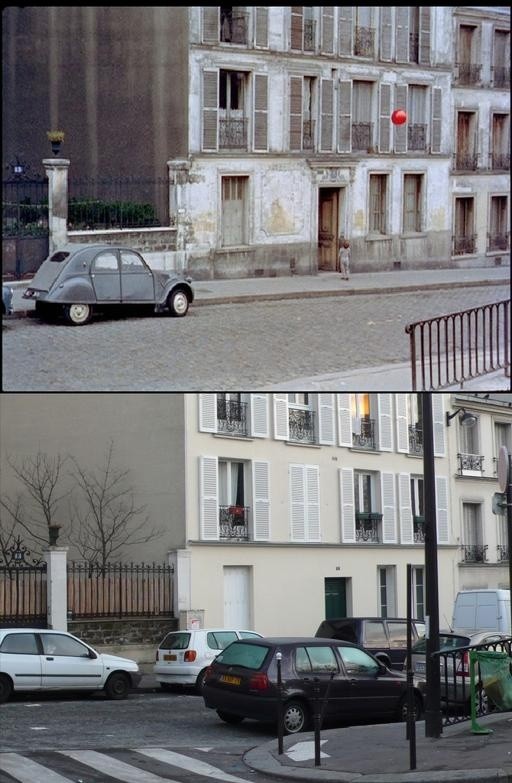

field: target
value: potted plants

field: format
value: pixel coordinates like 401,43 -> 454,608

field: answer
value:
47,525 -> 63,545
46,129 -> 66,158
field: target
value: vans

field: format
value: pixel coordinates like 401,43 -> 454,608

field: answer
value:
315,616 -> 429,669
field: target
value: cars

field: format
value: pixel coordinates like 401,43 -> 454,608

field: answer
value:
402,631 -> 512,717
23,244 -> 196,323
154,628 -> 264,692
0,628 -> 145,701
202,638 -> 421,733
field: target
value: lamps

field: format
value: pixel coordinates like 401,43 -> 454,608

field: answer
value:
446,405 -> 478,431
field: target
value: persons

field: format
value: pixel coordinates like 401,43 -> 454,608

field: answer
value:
338,240 -> 352,280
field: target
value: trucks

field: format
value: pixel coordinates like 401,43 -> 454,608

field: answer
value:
451,589 -> 511,635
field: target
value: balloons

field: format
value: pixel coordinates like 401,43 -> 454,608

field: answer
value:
390,108 -> 408,125
